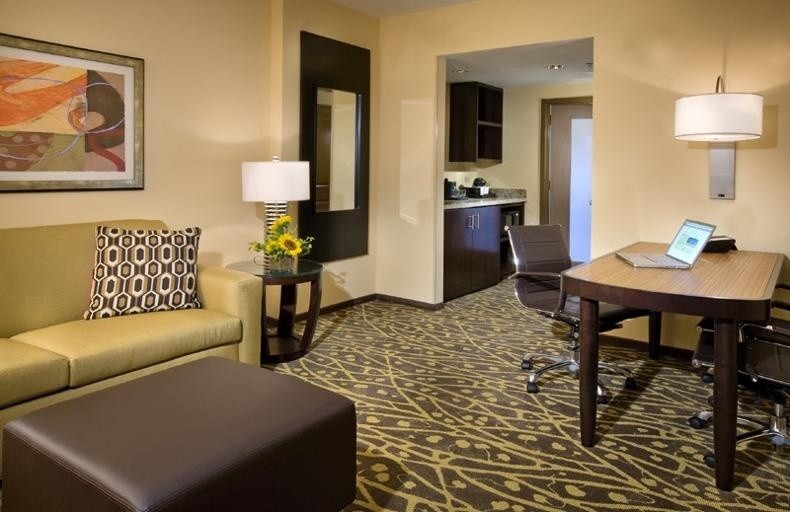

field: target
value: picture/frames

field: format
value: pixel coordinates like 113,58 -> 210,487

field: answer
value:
254,252 -> 294,273
0,32 -> 145,196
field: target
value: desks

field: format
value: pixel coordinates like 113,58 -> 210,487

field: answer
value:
560,240 -> 784,491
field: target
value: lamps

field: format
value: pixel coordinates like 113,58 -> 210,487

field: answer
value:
672,74 -> 767,202
238,156 -> 311,266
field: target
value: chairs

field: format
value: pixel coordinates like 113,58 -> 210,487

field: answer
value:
506,221 -> 655,403
685,279 -> 789,458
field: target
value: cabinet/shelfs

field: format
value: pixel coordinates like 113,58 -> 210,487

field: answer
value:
444,206 -> 502,302
450,80 -> 503,164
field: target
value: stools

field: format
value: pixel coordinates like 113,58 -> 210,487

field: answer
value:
225,259 -> 324,364
0,355 -> 358,511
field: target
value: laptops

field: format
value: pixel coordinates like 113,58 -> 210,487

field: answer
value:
615,219 -> 716,270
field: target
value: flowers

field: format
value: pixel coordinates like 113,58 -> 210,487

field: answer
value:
244,214 -> 305,264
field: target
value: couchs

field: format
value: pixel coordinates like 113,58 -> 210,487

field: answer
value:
0,217 -> 265,425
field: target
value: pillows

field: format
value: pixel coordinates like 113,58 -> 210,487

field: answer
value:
84,225 -> 204,320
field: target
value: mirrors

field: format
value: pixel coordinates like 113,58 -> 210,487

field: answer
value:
299,28 -> 370,269
